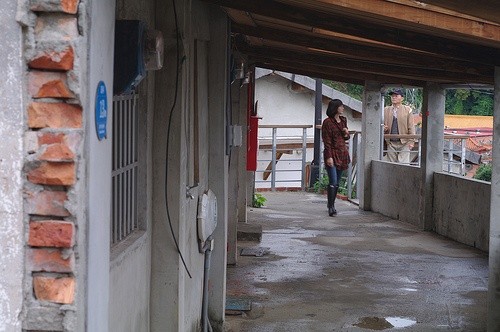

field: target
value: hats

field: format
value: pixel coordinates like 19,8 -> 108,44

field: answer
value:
388,89 -> 403,97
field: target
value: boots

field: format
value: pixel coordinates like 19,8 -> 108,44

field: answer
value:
333,186 -> 338,213
328,185 -> 334,216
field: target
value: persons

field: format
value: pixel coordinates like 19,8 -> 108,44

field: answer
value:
322,99 -> 351,216
382,90 -> 415,165
